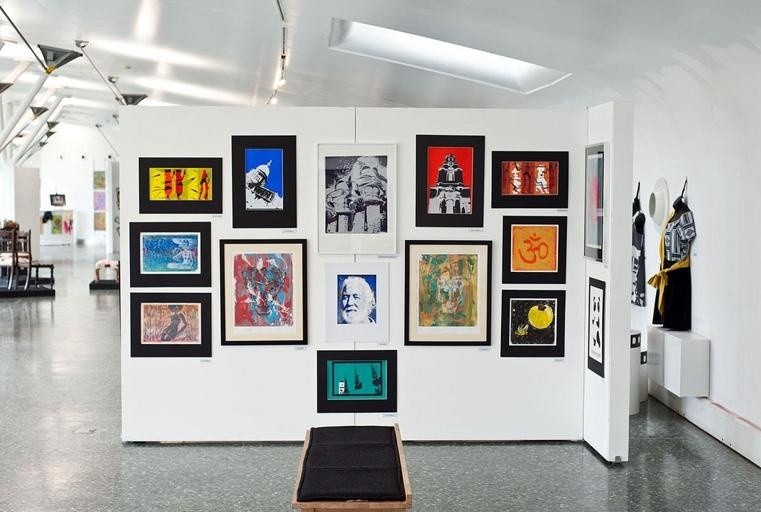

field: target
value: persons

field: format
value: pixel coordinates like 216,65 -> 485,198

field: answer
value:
632,199 -> 649,305
62,210 -> 73,247
649,198 -> 695,332
42,212 -> 55,235
337,275 -> 375,323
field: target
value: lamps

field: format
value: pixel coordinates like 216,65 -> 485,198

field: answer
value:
75,41 -> 148,106
0,6 -> 83,75
0,84 -> 59,158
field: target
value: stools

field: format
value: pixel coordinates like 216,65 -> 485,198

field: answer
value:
95,260 -> 119,283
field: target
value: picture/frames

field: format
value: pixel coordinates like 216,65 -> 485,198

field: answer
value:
404,239 -> 490,347
131,292 -> 212,358
232,136 -> 297,228
492,151 -> 568,209
415,135 -> 485,228
324,262 -> 390,344
218,238 -> 308,346
130,223 -> 212,287
502,289 -> 565,358
588,277 -> 605,378
583,143 -> 604,262
317,350 -> 397,413
138,157 -> 224,215
503,216 -> 567,285
316,142 -> 397,256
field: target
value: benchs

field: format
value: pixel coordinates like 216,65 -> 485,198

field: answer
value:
292,424 -> 413,512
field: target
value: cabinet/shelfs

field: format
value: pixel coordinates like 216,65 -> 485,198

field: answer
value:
647,324 -> 709,398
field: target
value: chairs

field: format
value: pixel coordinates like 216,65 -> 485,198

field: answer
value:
0,229 -> 53,292
